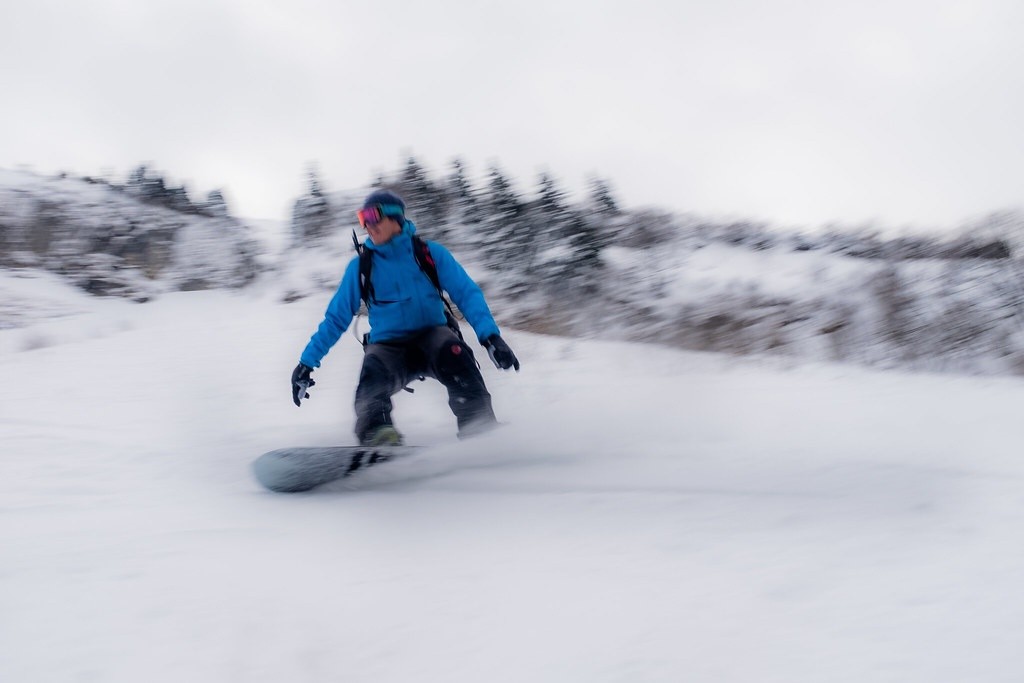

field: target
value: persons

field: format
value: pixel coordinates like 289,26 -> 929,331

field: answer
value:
291,191 -> 519,447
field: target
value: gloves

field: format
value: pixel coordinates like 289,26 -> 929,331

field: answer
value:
484,334 -> 519,370
291,362 -> 315,407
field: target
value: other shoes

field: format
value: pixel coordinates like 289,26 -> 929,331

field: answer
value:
365,426 -> 399,446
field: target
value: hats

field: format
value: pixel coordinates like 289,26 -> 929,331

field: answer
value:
363,191 -> 406,222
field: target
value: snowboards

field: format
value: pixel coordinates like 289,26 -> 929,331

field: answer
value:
255,447 -> 435,493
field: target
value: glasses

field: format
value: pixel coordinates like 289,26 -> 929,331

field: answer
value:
357,206 -> 382,229
